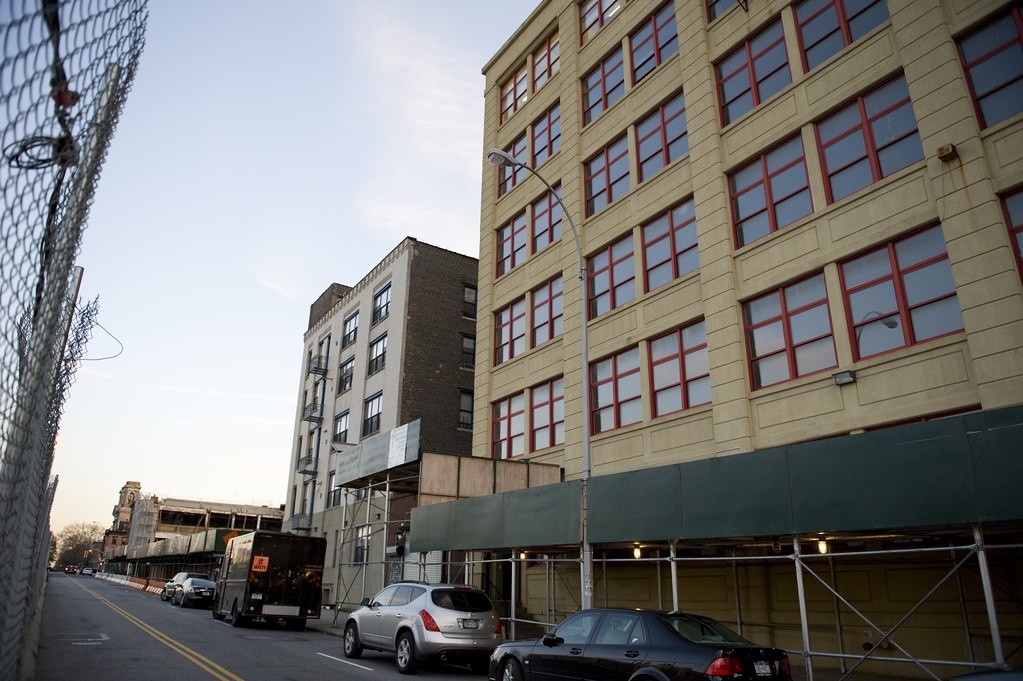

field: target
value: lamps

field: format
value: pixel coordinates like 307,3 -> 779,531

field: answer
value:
817,535 -> 828,553
632,544 -> 641,558
519,549 -> 525,559
832,370 -> 856,385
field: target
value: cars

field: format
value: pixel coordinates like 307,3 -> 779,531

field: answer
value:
171,578 -> 217,608
487,605 -> 793,681
47,562 -> 102,576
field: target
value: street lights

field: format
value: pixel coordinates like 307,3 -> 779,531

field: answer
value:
132,489 -> 148,579
487,145 -> 597,639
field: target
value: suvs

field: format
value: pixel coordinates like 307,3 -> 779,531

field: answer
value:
342,579 -> 504,675
161,571 -> 213,602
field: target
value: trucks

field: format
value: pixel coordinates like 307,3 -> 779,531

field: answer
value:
210,529 -> 328,629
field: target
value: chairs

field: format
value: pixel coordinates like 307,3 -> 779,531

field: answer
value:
604,619 -> 628,644
